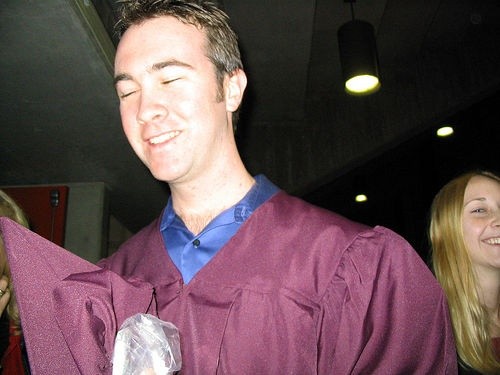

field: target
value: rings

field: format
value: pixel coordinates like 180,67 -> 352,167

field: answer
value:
0,289 -> 5,296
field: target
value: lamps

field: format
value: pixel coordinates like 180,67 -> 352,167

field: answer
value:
336,0 -> 384,96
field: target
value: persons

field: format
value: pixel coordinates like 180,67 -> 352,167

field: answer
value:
0,185 -> 32,375
92,0 -> 460,375
429,171 -> 500,375
